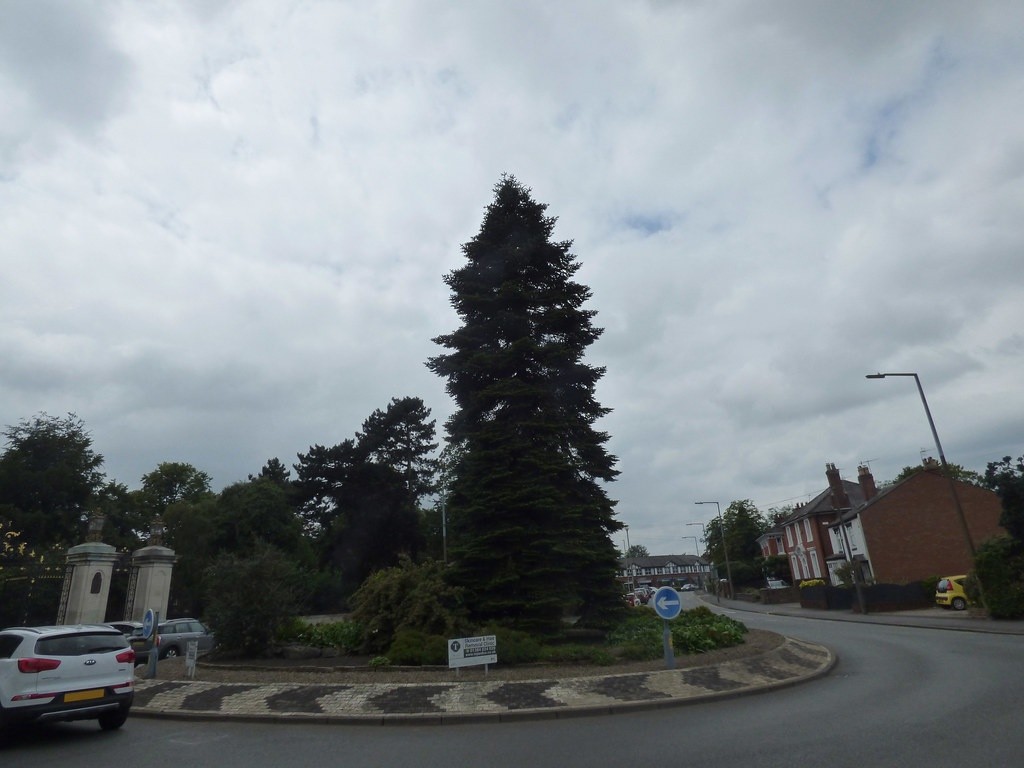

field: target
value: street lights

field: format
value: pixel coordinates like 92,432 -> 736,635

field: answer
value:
682,536 -> 701,574
686,522 -> 711,574
625,527 -> 637,590
695,501 -> 736,600
865,372 -> 993,616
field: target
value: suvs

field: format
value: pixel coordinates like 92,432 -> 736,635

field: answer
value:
128,616 -> 218,667
0,621 -> 136,743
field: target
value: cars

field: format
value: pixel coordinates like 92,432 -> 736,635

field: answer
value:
680,584 -> 695,591
766,579 -> 791,590
934,575 -> 978,611
106,620 -> 143,636
623,583 -> 658,608
